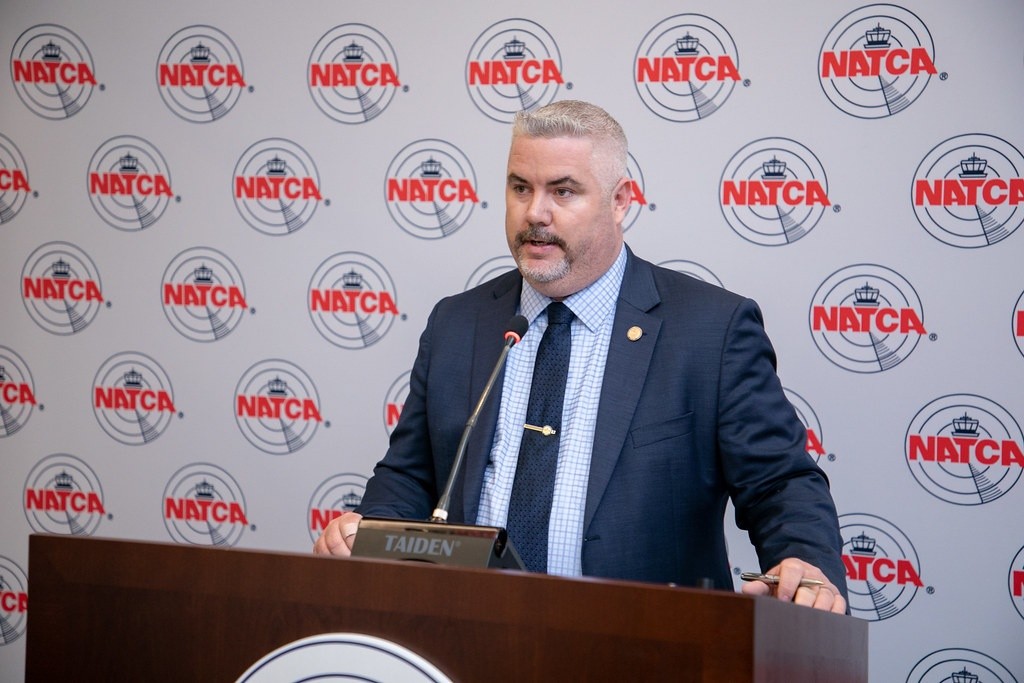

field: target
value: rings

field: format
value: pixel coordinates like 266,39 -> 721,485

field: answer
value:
820,586 -> 834,595
343,523 -> 358,538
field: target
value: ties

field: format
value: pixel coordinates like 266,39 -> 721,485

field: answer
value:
505,304 -> 574,575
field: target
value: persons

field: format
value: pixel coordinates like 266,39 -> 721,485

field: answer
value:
311,99 -> 850,620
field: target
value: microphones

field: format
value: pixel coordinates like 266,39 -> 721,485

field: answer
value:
349,315 -> 529,570
